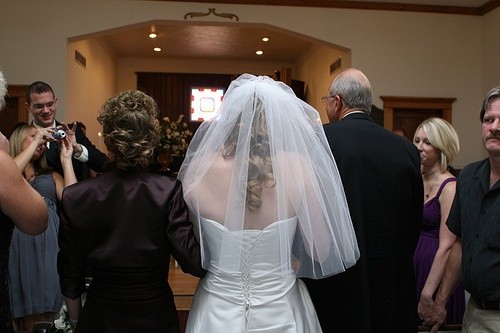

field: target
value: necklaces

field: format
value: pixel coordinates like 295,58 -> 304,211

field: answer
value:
425,168 -> 440,199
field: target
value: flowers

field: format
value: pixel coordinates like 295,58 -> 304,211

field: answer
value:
54,302 -> 72,333
157,114 -> 193,158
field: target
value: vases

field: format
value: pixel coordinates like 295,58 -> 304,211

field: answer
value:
157,152 -> 174,172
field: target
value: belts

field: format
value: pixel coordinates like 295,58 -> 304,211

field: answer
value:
471,292 -> 500,311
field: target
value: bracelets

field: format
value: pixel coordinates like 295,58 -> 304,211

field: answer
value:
70,319 -> 77,323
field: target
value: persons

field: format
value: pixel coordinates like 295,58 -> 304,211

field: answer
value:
423,88 -> 500,333
414,118 -> 466,332
290,68 -> 424,333
177,74 -> 360,333
57,89 -> 208,333
0,72 -> 115,332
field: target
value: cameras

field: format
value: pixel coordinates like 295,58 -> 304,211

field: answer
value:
46,128 -> 65,140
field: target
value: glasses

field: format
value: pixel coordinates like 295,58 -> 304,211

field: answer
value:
321,96 -> 337,103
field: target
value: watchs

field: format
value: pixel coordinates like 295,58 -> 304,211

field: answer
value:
73,147 -> 83,158
